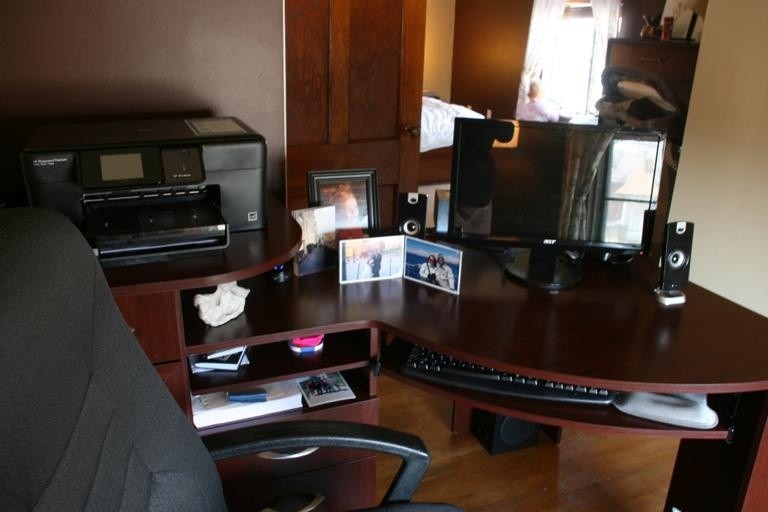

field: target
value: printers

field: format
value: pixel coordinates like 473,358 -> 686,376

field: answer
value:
19,114 -> 264,271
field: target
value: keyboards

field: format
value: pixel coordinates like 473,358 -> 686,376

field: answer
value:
402,343 -> 616,404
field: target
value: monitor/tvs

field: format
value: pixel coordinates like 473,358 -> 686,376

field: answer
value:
450,116 -> 669,294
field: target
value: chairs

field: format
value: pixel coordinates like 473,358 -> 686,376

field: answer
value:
0,204 -> 463,512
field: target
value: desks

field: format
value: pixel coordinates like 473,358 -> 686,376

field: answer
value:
379,233 -> 768,512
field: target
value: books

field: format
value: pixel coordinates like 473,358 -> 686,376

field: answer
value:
187,345 -> 305,432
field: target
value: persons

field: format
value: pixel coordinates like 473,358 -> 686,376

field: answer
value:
520,78 -> 559,123
324,183 -> 360,228
419,254 -> 437,282
366,248 -> 382,277
306,377 -> 342,398
431,253 -> 456,290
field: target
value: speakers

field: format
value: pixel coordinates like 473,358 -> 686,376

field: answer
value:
657,222 -> 694,308
471,411 -> 540,457
397,191 -> 427,240
